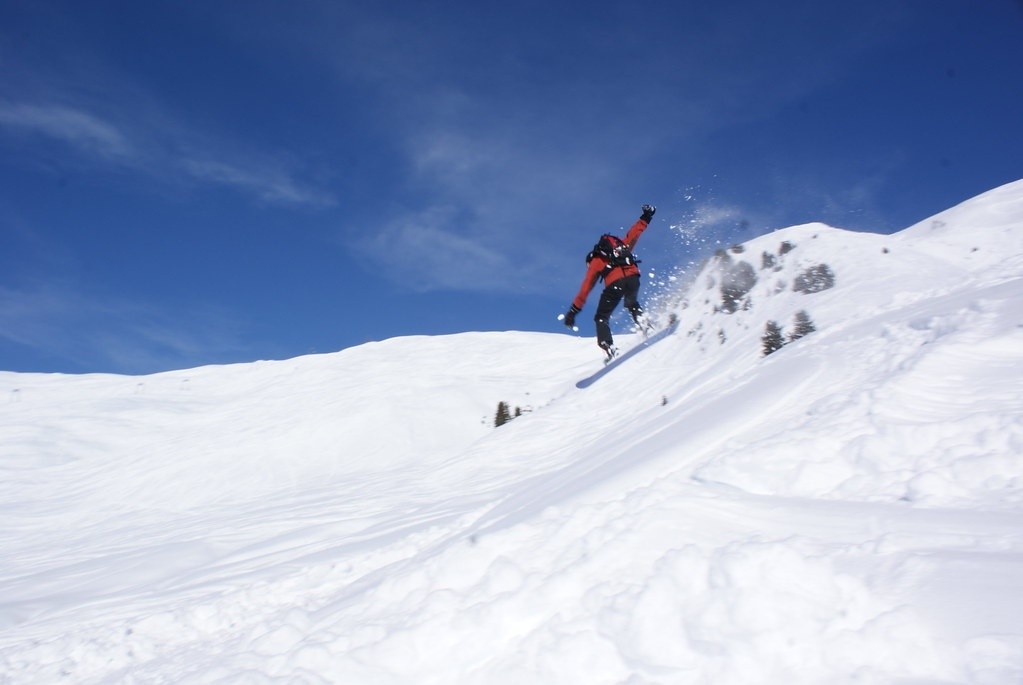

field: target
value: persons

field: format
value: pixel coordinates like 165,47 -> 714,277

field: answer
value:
565,204 -> 656,362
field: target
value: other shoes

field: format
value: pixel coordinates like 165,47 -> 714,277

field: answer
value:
601,341 -> 621,365
638,319 -> 657,339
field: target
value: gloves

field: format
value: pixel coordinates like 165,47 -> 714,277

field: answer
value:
640,205 -> 656,224
563,304 -> 582,327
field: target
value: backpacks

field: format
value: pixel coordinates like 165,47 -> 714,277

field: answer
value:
594,232 -> 641,283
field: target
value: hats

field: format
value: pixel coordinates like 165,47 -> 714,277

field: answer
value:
586,251 -> 595,263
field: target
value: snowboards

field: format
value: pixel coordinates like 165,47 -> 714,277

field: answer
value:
576,318 -> 682,390
